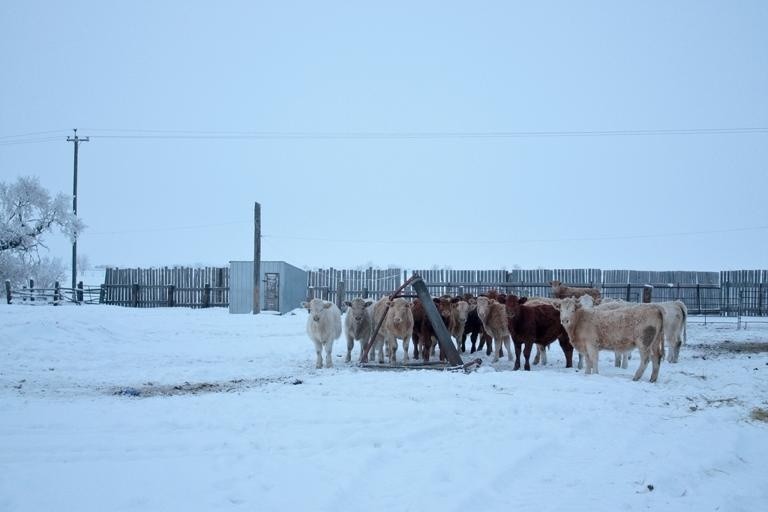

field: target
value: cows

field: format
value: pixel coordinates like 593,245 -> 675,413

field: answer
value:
547,280 -> 601,298
300,297 -> 342,370
